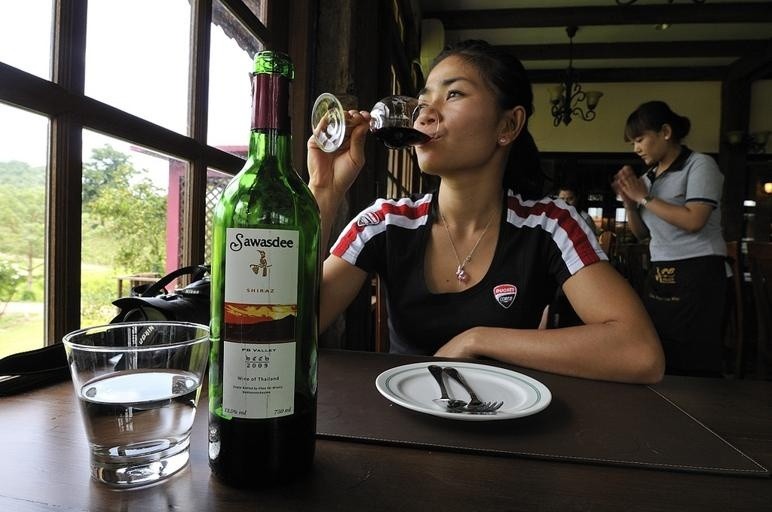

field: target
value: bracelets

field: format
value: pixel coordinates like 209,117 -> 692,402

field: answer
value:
623,203 -> 639,211
641,194 -> 655,206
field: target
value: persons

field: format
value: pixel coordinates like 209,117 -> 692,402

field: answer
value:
612,100 -> 729,377
305,39 -> 666,385
555,187 -> 596,237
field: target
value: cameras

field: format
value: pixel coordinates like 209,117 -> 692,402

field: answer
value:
104,260 -> 214,409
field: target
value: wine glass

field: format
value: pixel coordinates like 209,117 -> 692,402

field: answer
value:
311,92 -> 440,153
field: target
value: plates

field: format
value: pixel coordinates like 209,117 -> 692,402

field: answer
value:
373,361 -> 551,420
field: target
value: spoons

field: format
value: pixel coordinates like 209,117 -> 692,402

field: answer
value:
425,365 -> 467,413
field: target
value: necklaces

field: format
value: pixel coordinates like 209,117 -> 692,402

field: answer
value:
437,192 -> 504,283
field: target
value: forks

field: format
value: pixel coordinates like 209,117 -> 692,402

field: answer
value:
443,366 -> 503,411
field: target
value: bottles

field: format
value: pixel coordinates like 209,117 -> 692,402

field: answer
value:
206,47 -> 323,493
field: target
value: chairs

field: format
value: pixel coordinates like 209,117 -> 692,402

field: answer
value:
373,273 -> 557,356
725,239 -> 749,382
747,241 -> 772,381
609,243 -> 652,299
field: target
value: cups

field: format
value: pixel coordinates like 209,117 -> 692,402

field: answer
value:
62,320 -> 212,492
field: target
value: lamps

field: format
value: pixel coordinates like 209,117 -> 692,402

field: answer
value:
544,27 -> 604,129
